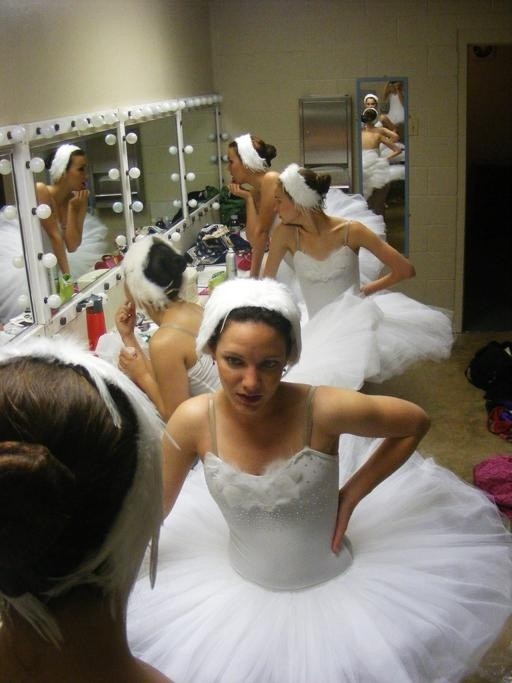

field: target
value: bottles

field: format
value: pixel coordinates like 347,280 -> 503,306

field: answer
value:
156,217 -> 164,229
229,215 -> 239,233
86,300 -> 106,350
225,248 -> 236,281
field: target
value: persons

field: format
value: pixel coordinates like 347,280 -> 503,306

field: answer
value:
262,162 -> 457,401
360,107 -> 401,214
223,133 -> 388,283
0,139 -> 110,327
380,80 -> 405,129
116,232 -> 385,432
362,92 -> 395,136
1,336 -> 174,682
126,275 -> 512,683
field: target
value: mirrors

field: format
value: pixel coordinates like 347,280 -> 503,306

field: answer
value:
357,76 -> 410,257
0,105 -> 220,351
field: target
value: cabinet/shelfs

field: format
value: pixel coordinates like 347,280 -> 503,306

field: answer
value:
297,96 -> 351,165
